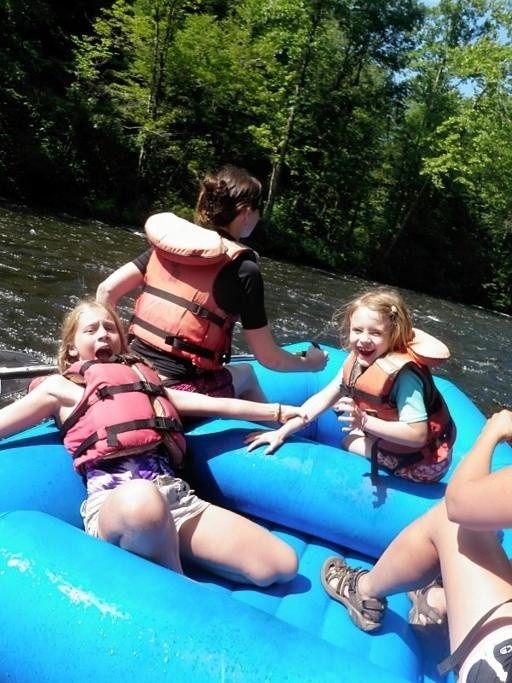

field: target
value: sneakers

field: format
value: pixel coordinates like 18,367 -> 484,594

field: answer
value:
406,578 -> 444,639
321,551 -> 387,636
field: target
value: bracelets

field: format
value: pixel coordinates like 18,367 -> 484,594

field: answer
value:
361,411 -> 366,428
274,402 -> 280,423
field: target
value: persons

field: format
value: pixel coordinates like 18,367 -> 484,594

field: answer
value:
242,286 -> 457,483
0,302 -> 308,589
95,163 -> 329,441
321,409 -> 512,683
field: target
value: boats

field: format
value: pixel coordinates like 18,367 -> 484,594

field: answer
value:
0,341 -> 512,682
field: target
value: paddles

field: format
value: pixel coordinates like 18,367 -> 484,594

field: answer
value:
0,340 -> 325,397
